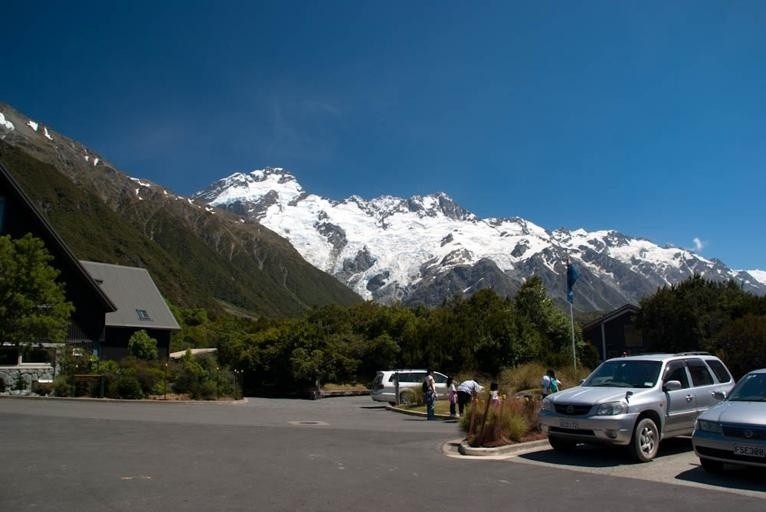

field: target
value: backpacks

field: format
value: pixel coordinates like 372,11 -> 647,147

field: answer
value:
422,381 -> 431,393
544,376 -> 558,396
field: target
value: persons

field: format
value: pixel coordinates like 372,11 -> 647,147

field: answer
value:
457,377 -> 480,417
540,368 -> 562,399
446,376 -> 460,418
489,382 -> 502,408
423,367 -> 440,421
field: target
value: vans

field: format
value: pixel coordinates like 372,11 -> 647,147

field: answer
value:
372,366 -> 461,407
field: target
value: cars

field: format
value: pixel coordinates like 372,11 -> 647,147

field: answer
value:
692,368 -> 766,479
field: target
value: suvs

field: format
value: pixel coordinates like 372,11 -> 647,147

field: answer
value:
537,350 -> 738,462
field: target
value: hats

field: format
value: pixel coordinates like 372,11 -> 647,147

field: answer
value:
427,366 -> 435,371
623,351 -> 628,355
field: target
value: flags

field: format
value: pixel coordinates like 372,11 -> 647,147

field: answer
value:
566,263 -> 578,305
